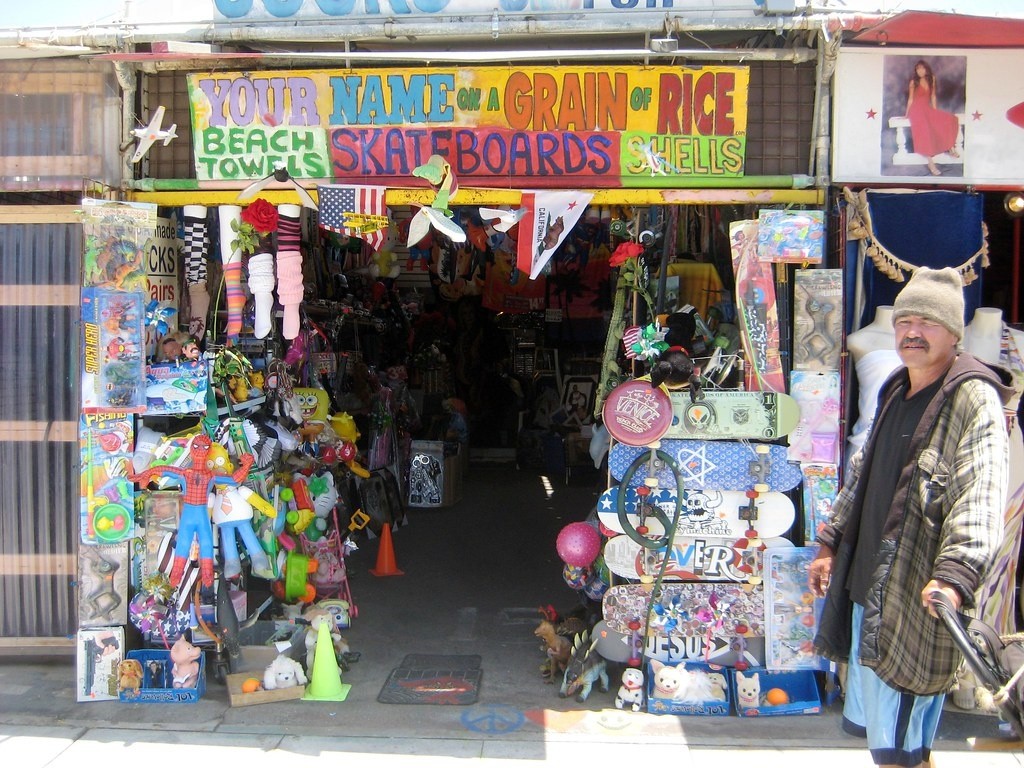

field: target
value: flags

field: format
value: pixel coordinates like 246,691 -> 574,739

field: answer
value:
316,185 -> 387,255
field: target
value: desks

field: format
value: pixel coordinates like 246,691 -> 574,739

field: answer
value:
563,432 -> 607,486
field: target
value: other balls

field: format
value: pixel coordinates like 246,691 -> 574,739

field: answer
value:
555,521 -> 610,603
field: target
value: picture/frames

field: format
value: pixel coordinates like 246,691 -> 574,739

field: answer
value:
557,374 -> 599,427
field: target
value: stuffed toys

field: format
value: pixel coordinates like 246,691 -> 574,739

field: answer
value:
262,654 -> 308,691
302,608 -> 342,682
650,311 -> 706,405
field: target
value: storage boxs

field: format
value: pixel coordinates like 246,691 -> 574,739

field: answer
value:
76,591 -> 305,708
408,440 -> 469,509
730,668 -> 821,718
645,658 -> 730,717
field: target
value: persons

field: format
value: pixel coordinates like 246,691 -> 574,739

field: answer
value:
839,305 -> 904,704
895,58 -> 961,176
808,266 -> 1010,768
953,307 -> 1024,713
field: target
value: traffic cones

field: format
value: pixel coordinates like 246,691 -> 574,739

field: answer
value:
299,620 -> 353,702
369,522 -> 405,576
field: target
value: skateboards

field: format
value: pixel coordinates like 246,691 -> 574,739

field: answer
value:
590,387 -> 805,670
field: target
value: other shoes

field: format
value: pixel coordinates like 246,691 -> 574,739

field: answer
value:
948,146 -> 960,158
927,163 -> 942,176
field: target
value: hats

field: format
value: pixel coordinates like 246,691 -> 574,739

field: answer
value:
891,266 -> 965,342
